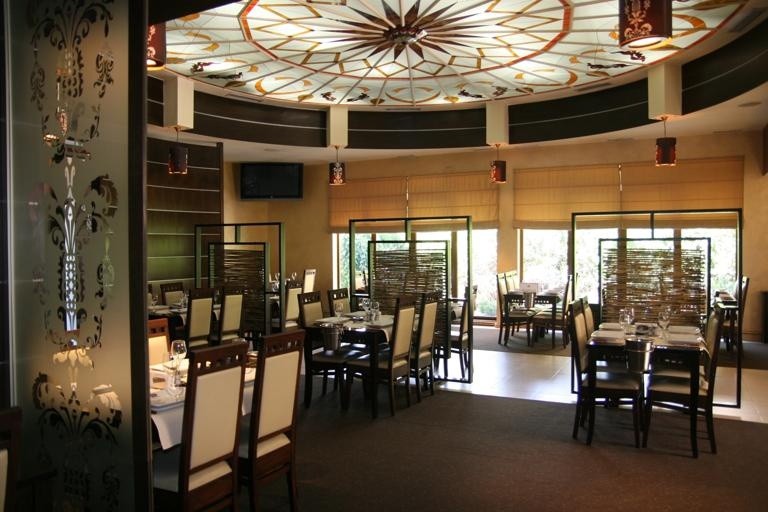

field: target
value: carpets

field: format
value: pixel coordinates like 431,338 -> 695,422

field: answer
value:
146,360 -> 768,512
448,322 -> 768,372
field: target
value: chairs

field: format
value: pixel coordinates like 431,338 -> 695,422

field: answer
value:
562,294 -> 726,460
149,339 -> 251,512
708,274 -> 751,354
281,265 -> 479,420
491,264 -> 579,351
223,327 -> 306,510
147,279 -> 265,361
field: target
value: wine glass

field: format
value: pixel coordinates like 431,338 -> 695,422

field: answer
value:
334,303 -> 343,322
362,299 -> 381,322
170,340 -> 187,384
658,310 -> 670,338
619,306 -> 634,336
290,272 -> 297,285
150,295 -> 159,312
275,272 -> 280,282
165,352 -> 181,389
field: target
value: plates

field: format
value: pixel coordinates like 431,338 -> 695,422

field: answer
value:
147,386 -> 187,411
667,324 -> 701,335
545,290 -> 560,295
316,310 -> 394,327
170,308 -> 187,312
598,322 -> 622,330
591,330 -> 625,339
668,333 -> 702,345
243,367 -> 256,386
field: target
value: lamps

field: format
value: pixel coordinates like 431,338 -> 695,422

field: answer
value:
146,20 -> 167,68
617,0 -> 674,52
487,144 -> 507,184
653,116 -> 678,167
166,126 -> 189,176
327,145 -> 347,187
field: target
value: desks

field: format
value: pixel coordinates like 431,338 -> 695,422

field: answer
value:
148,357 -> 292,451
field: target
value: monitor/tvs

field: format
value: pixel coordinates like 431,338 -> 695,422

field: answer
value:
238,162 -> 304,201
